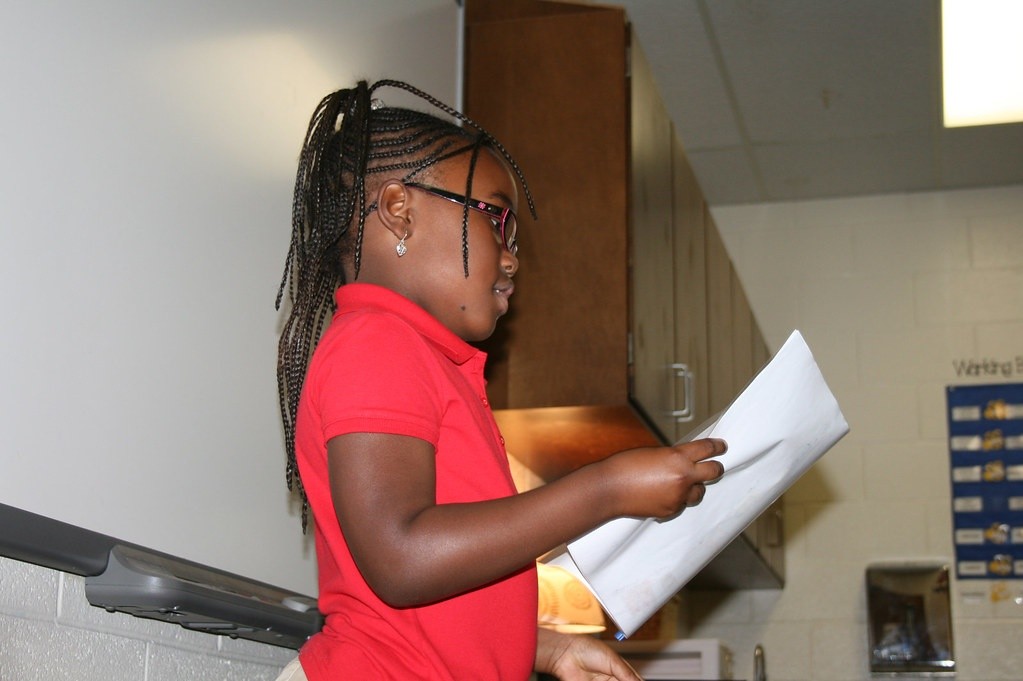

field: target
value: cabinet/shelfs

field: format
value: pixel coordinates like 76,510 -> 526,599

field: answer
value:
460,0 -> 786,589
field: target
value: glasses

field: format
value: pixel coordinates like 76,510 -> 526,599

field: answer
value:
370,181 -> 518,256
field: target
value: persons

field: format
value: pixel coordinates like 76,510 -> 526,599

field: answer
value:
274,79 -> 727,681
